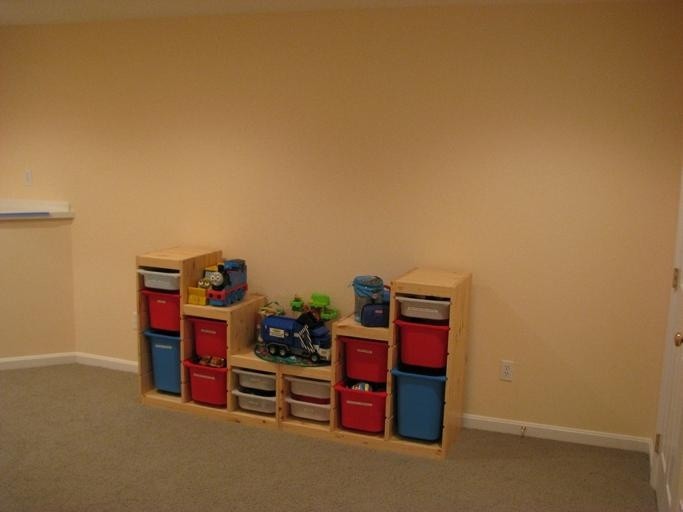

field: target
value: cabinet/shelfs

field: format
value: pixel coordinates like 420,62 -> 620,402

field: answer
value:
135,244 -> 472,461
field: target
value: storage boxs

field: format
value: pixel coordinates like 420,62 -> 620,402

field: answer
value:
390,364 -> 447,440
284,375 -> 330,399
184,357 -> 227,404
136,268 -> 181,290
394,296 -> 451,320
143,329 -> 181,393
231,387 -> 276,413
334,378 -> 386,432
336,336 -> 388,383
142,289 -> 181,331
284,393 -> 330,421
231,368 -> 276,391
394,318 -> 449,368
187,317 -> 227,361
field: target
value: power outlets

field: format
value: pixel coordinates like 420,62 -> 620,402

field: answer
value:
498,358 -> 514,383
